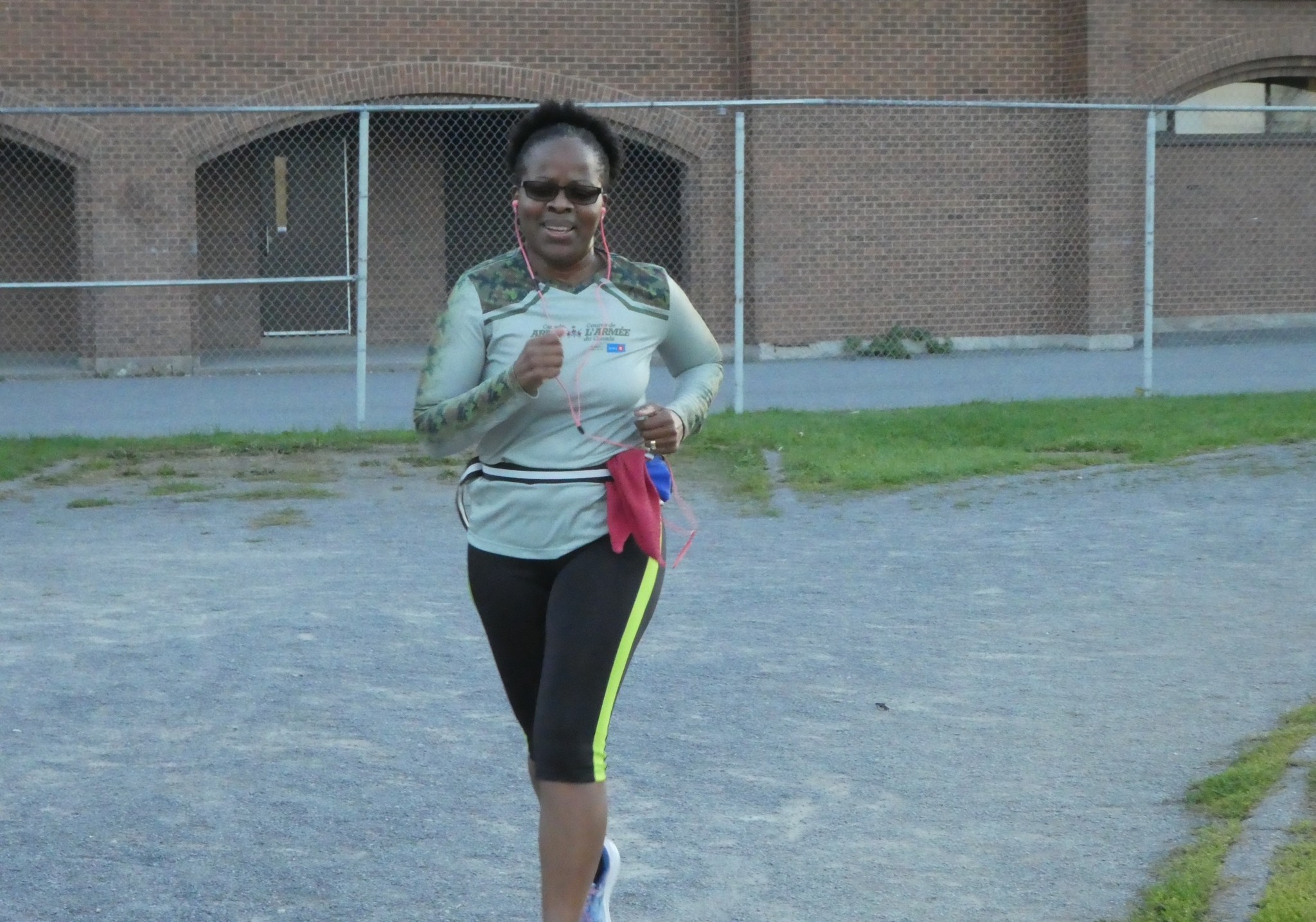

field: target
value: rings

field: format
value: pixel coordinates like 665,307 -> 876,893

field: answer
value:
649,439 -> 657,451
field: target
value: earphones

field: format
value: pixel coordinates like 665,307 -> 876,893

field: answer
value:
512,200 -> 518,212
600,207 -> 607,220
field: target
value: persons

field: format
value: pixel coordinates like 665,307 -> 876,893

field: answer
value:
412,97 -> 724,922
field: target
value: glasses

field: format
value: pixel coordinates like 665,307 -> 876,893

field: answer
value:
522,181 -> 604,206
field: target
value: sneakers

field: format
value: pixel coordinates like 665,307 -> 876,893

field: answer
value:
582,839 -> 619,922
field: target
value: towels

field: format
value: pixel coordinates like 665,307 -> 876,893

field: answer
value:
605,447 -> 667,568
